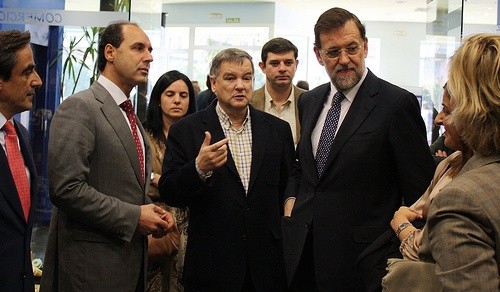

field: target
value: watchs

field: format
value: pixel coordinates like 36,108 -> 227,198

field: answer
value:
395,221 -> 412,238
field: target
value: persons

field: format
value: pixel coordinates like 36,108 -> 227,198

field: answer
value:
195,75 -> 216,111
139,70 -> 198,292
41,21 -> 176,292
0,30 -> 43,292
296,7 -> 438,292
297,80 -> 309,91
390,83 -> 474,261
381,32 -> 500,292
250,37 -> 308,153
157,47 -> 297,292
192,81 -> 201,94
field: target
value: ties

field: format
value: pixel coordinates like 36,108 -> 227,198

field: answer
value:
3,121 -> 30,223
120,99 -> 144,184
315,91 -> 346,178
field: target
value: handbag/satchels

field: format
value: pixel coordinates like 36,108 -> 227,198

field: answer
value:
147,139 -> 181,260
